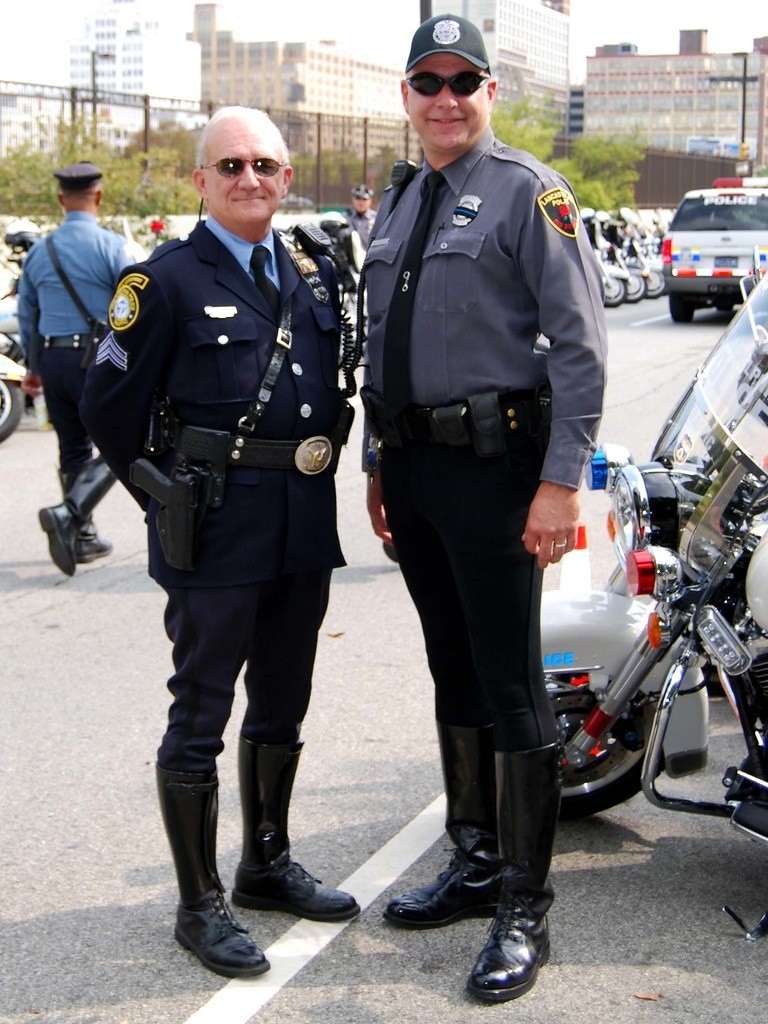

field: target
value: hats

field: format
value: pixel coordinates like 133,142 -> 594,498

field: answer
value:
405,13 -> 492,77
352,186 -> 374,200
53,162 -> 104,191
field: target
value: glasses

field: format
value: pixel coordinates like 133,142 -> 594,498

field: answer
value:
201,157 -> 287,177
406,71 -> 490,96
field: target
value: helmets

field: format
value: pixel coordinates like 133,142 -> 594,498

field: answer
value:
4,219 -> 41,246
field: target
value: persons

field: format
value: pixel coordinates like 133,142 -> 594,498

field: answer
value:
359,12 -> 607,1000
344,187 -> 377,252
18,163 -> 137,578
78,104 -> 363,979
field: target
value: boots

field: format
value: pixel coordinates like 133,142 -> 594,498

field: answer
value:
467,740 -> 564,1001
58,469 -> 113,564
230,733 -> 360,922
382,723 -> 503,928
154,763 -> 271,977
39,455 -> 117,576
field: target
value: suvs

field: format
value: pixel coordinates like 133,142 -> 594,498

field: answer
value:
658,176 -> 768,323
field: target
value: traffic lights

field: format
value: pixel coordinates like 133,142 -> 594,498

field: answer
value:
740,142 -> 749,159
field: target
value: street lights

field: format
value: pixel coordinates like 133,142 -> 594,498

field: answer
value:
731,51 -> 749,161
90,50 -> 113,151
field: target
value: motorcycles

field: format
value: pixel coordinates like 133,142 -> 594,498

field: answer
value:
0,218 -> 44,446
539,266 -> 768,950
577,207 -> 679,307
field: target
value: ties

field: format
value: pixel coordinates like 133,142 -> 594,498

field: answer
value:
250,245 -> 281,324
381,170 -> 447,422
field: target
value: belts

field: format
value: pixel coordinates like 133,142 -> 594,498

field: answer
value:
49,333 -> 90,348
226,435 -> 333,475
393,403 -> 529,446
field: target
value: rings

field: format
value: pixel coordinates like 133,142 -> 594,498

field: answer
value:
554,544 -> 566,548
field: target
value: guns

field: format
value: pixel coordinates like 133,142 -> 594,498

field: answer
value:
538,386 -> 553,449
128,457 -> 198,574
